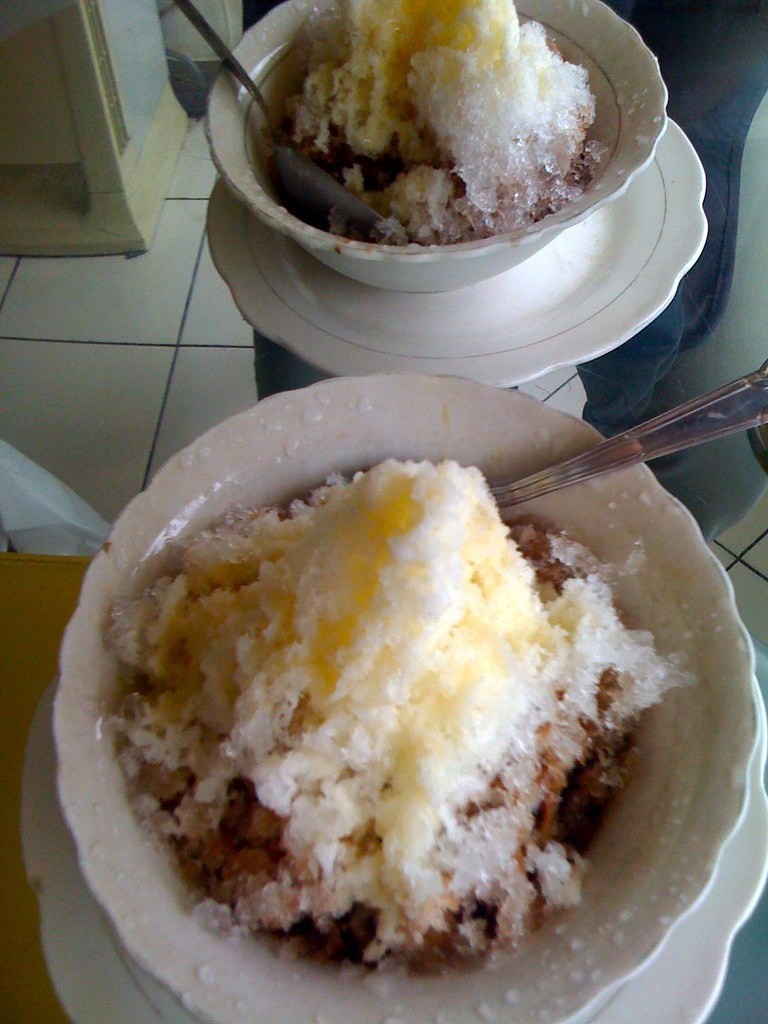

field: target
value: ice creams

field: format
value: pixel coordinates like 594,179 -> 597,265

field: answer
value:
104,456 -> 700,982
261,0 -> 610,248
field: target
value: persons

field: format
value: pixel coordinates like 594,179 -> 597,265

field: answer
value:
243,0 -> 768,484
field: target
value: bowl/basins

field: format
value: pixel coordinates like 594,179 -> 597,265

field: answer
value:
197,2 -> 673,294
50,371 -> 762,1024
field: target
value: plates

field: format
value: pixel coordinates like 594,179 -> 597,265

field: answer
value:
20,655 -> 767,1024
206,111 -> 710,379
